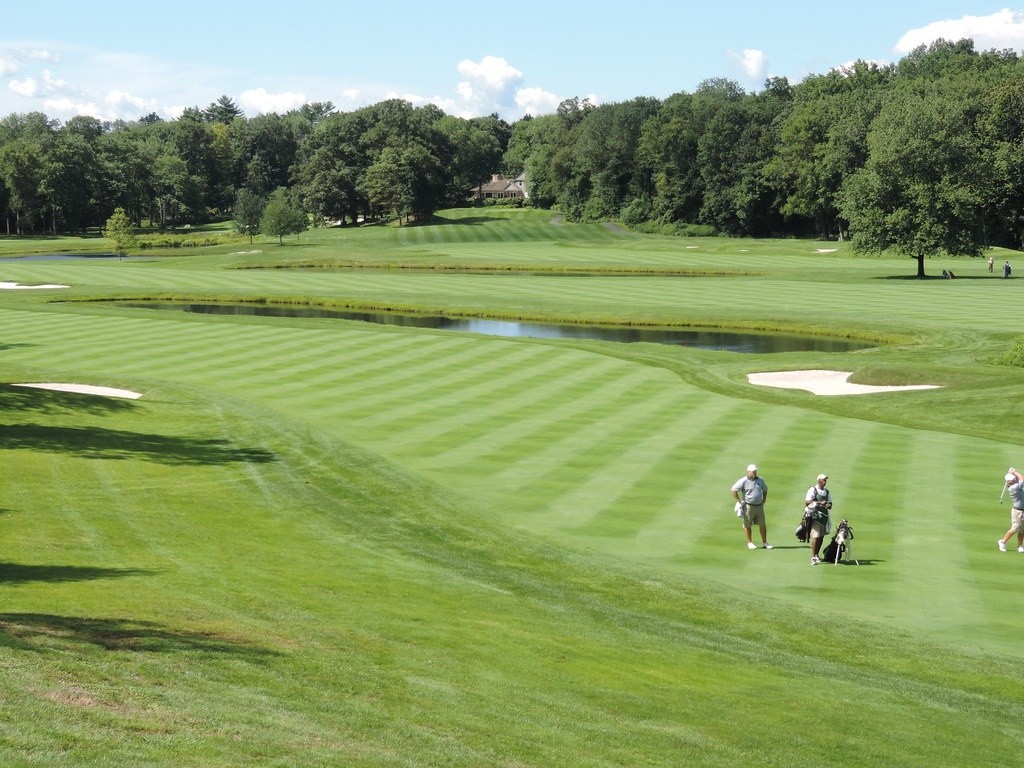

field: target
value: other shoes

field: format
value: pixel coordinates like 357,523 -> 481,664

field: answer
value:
763,543 -> 773,548
749,544 -> 757,549
816,557 -> 821,562
812,558 -> 816,564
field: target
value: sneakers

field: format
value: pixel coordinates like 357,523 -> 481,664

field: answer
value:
998,541 -> 1006,552
1017,547 -> 1024,552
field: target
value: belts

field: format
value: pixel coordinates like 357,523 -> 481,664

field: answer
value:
1013,507 -> 1024,510
746,503 -> 762,506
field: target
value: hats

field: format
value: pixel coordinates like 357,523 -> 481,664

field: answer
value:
1005,474 -> 1016,480
817,474 -> 828,480
747,465 -> 756,471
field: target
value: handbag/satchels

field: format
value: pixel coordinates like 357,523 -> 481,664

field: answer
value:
824,534 -> 845,561
825,516 -> 831,534
797,515 -> 813,541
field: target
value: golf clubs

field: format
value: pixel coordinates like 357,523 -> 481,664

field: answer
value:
999,467 -> 1013,504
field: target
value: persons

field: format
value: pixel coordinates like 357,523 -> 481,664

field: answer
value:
997,469 -> 1024,553
1002,261 -> 1011,278
942,269 -> 955,279
805,474 -> 832,565
731,464 -> 773,549
988,258 -> 993,272
